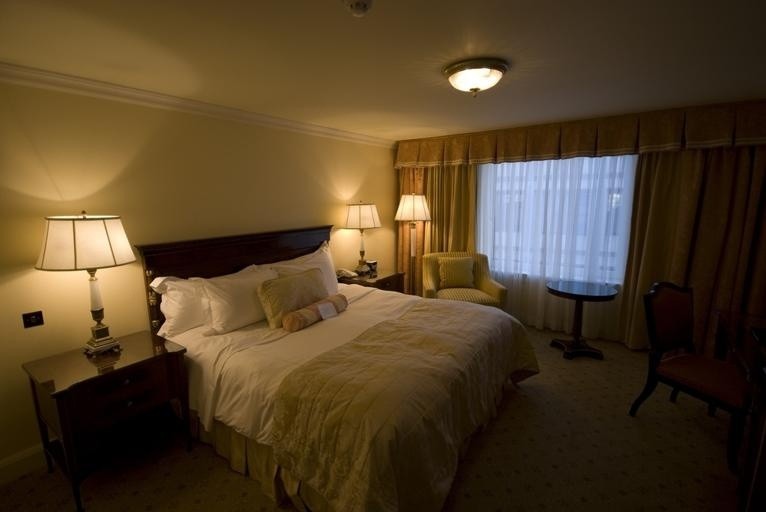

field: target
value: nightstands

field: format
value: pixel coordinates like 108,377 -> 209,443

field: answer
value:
338,271 -> 405,294
20,330 -> 193,512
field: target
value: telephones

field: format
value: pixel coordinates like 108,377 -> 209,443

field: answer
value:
336,268 -> 359,278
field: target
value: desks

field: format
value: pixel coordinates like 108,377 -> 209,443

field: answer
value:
545,281 -> 618,360
708,308 -> 766,511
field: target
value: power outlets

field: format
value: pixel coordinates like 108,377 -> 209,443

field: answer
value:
22,310 -> 44,328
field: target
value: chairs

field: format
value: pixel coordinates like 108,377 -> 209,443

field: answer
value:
629,280 -> 752,471
422,252 -> 508,311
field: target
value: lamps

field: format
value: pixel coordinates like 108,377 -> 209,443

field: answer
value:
33,209 -> 137,358
394,192 -> 432,295
442,59 -> 509,99
344,200 -> 382,275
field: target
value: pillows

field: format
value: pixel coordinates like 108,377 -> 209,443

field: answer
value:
271,244 -> 338,295
257,268 -> 329,329
254,240 -> 339,295
189,269 -> 279,336
282,294 -> 348,333
149,276 -> 204,338
437,256 -> 475,289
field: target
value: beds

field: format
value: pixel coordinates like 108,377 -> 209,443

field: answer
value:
132,225 -> 540,512
